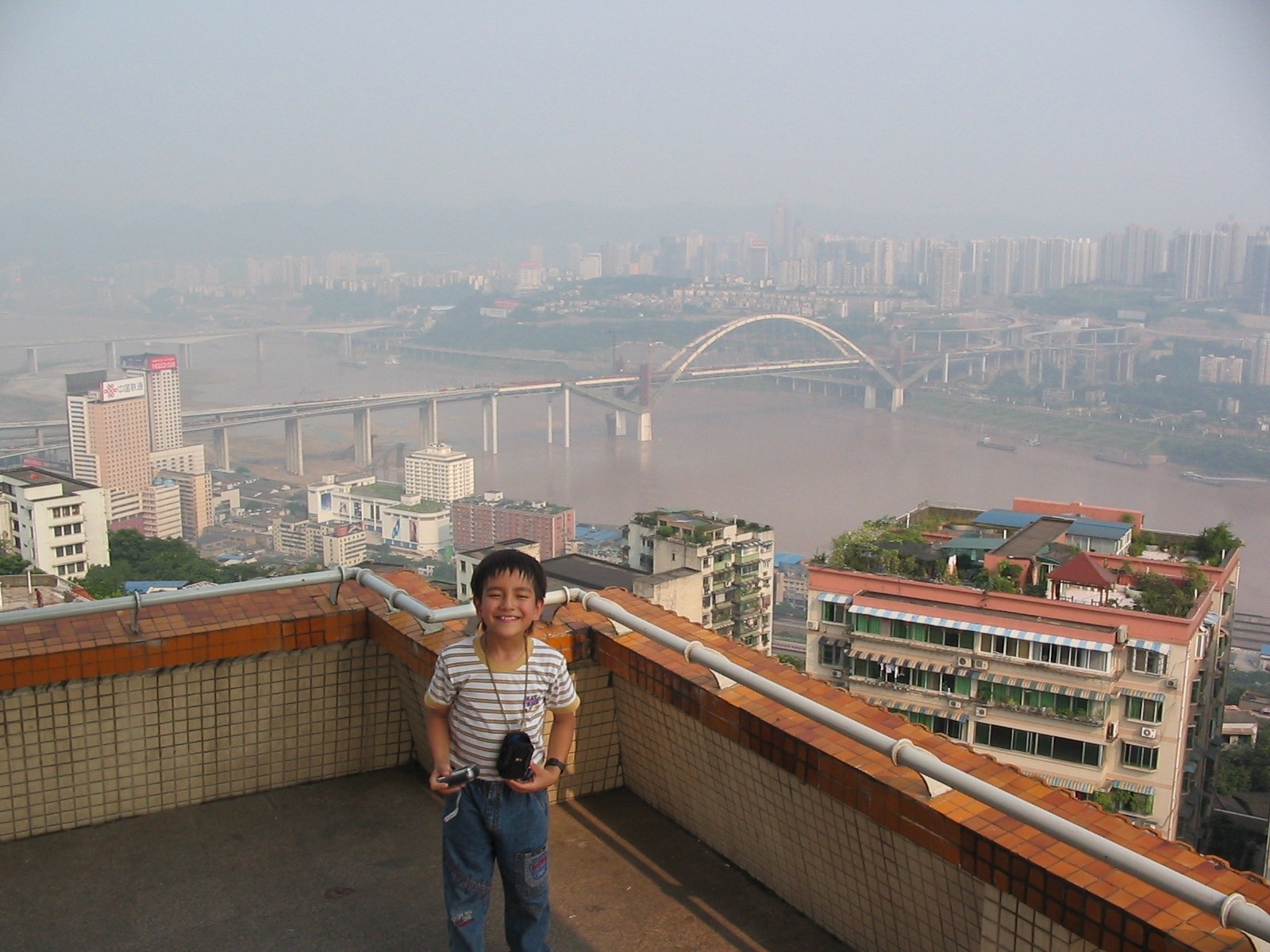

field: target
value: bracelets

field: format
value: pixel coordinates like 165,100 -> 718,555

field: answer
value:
543,758 -> 566,776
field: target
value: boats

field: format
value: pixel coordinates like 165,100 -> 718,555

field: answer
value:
1092,453 -> 1149,471
1179,470 -> 1226,489
976,435 -> 1016,451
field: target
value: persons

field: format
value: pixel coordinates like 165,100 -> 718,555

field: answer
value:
424,549 -> 581,952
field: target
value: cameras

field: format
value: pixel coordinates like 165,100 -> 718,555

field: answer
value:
438,763 -> 479,788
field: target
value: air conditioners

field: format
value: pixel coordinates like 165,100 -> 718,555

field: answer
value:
725,582 -> 731,587
1117,624 -> 1127,642
1109,722 -> 1118,737
1140,727 -> 1156,738
974,658 -> 988,670
831,669 -> 842,678
762,548 -> 766,553
1166,678 -> 1177,688
957,656 -> 972,667
806,620 -> 819,630
948,699 -> 961,708
760,608 -> 765,613
975,707 -> 987,717
1143,822 -> 1156,834
726,562 -> 732,567
